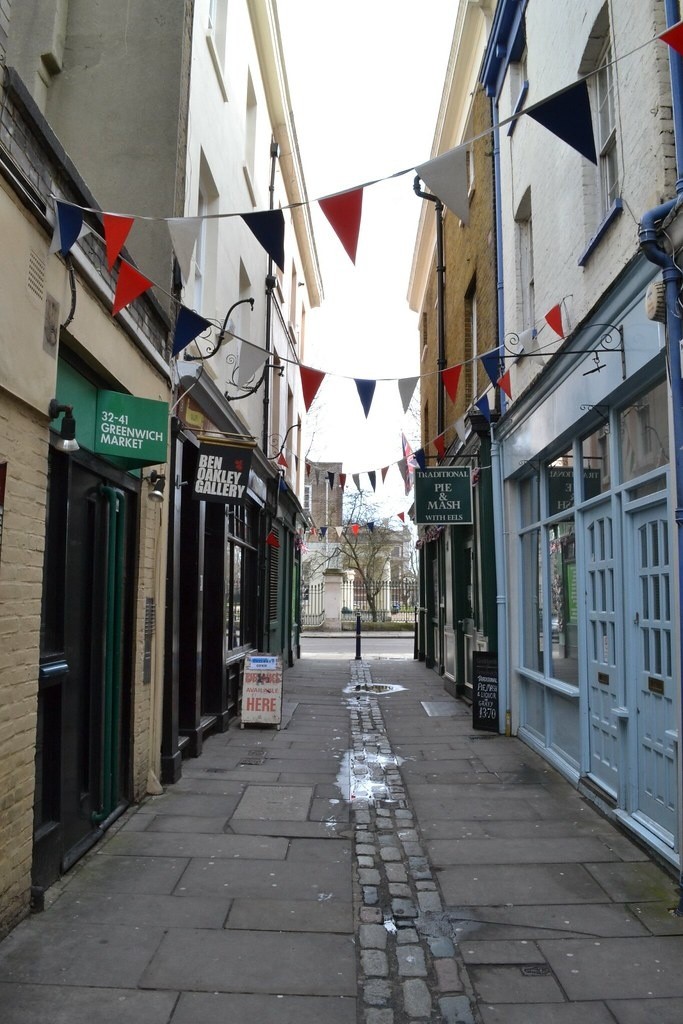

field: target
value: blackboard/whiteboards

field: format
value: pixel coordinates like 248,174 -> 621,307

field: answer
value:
473,650 -> 500,732
